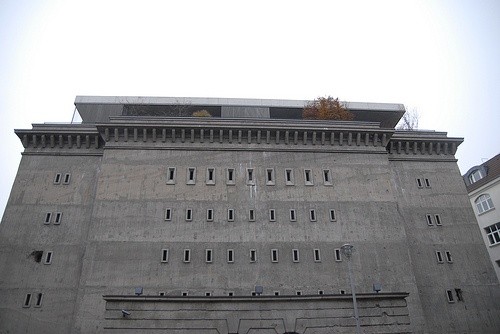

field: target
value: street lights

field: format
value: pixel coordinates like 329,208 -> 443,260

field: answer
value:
340,243 -> 361,334
